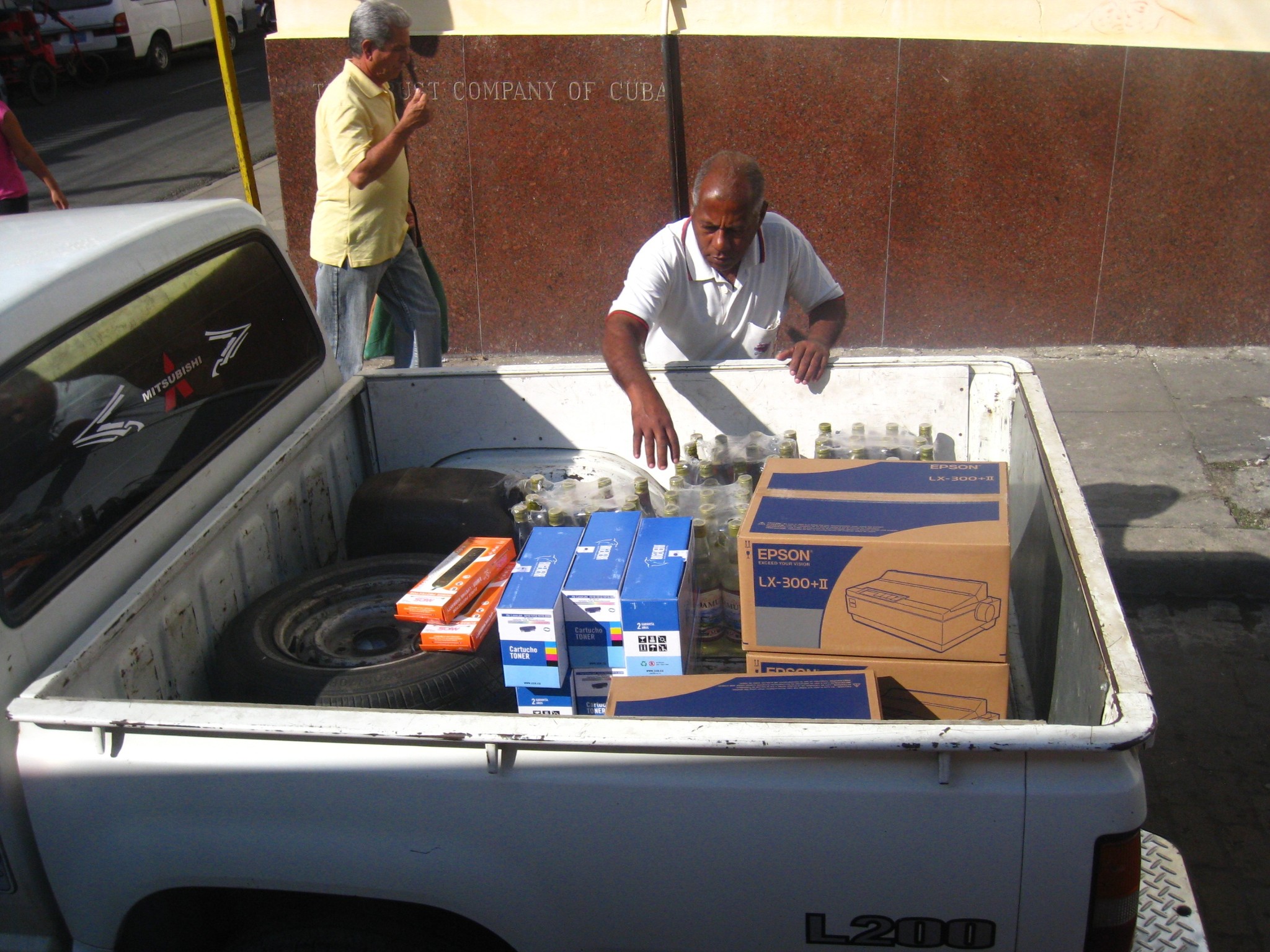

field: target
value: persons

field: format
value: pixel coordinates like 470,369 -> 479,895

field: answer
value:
0,97 -> 69,216
603,150 -> 848,470
306,4 -> 456,372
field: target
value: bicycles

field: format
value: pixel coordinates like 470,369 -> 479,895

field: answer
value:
260,2 -> 276,34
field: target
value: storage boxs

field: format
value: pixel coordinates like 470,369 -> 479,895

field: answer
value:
395,457 -> 1010,720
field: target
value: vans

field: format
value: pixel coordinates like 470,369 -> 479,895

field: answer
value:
31,0 -> 246,88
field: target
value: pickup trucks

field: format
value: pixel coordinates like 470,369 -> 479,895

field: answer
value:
1,197 -> 1212,952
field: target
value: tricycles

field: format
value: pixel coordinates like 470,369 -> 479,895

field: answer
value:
0,0 -> 109,107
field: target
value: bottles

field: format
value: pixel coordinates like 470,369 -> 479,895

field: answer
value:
510,422 -> 936,652
